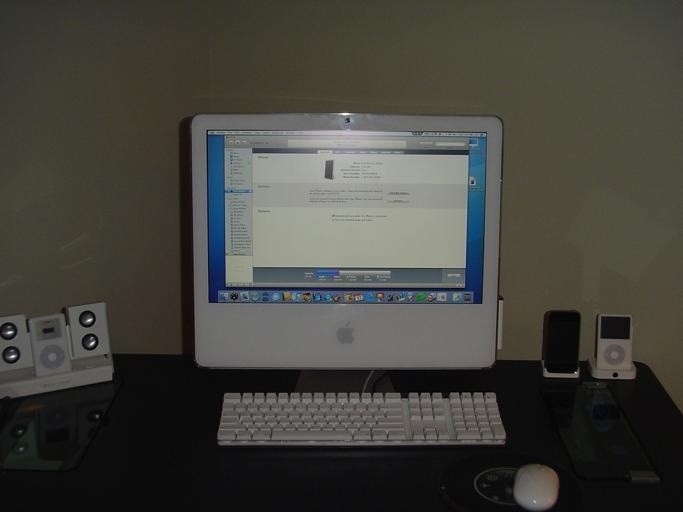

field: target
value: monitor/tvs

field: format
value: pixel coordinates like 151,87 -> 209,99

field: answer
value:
193,114 -> 504,393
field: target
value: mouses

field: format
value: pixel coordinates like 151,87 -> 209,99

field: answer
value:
513,464 -> 562,508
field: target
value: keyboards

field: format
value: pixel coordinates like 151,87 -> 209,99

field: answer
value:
219,391 -> 506,446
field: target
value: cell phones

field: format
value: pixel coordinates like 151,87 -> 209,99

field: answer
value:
546,308 -> 579,374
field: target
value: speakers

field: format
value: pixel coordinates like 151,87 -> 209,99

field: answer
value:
69,300 -> 109,360
0,313 -> 31,371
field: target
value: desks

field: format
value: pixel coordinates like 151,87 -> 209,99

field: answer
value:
1,355 -> 682,510
